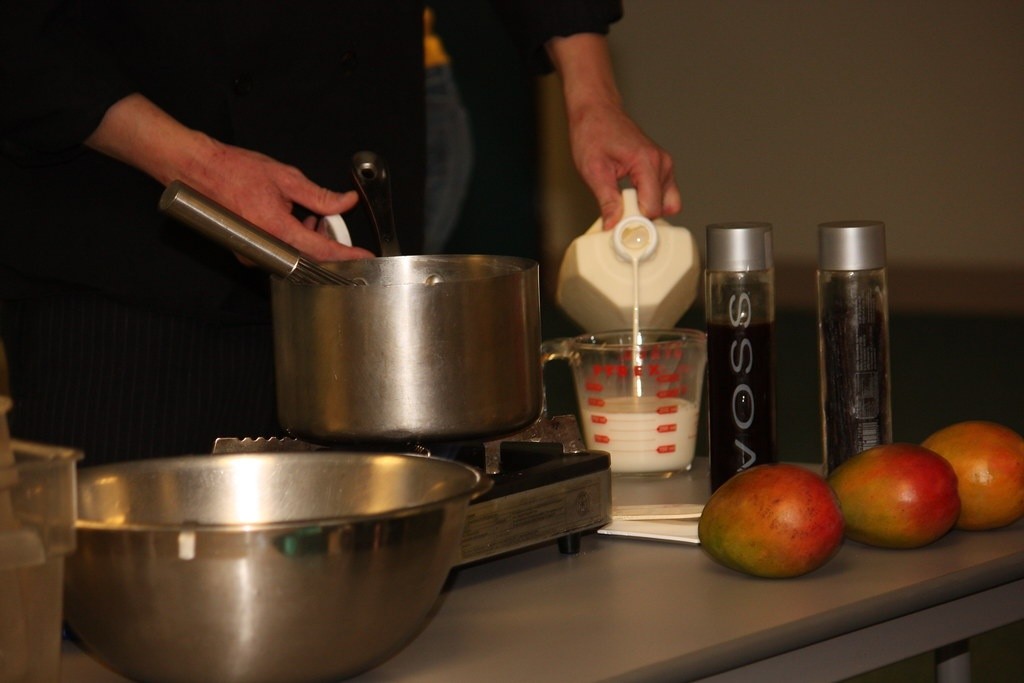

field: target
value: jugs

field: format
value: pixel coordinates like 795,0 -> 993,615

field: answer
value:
558,188 -> 702,343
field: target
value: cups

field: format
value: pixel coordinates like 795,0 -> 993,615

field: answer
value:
539,326 -> 705,481
0,438 -> 85,683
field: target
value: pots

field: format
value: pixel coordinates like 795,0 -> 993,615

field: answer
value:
269,253 -> 545,447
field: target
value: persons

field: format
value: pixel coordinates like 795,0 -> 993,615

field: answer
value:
1,0 -> 683,469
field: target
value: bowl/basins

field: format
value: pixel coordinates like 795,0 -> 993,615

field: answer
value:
18,452 -> 480,683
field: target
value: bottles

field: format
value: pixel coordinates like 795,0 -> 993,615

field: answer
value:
816,219 -> 894,478
703,221 -> 774,494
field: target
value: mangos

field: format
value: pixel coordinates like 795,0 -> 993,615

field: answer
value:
919,421 -> 1024,527
697,464 -> 848,579
829,445 -> 961,550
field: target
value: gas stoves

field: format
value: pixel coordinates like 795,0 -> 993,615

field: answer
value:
213,435 -> 614,573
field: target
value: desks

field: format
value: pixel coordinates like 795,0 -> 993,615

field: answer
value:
51,460 -> 1024,683
